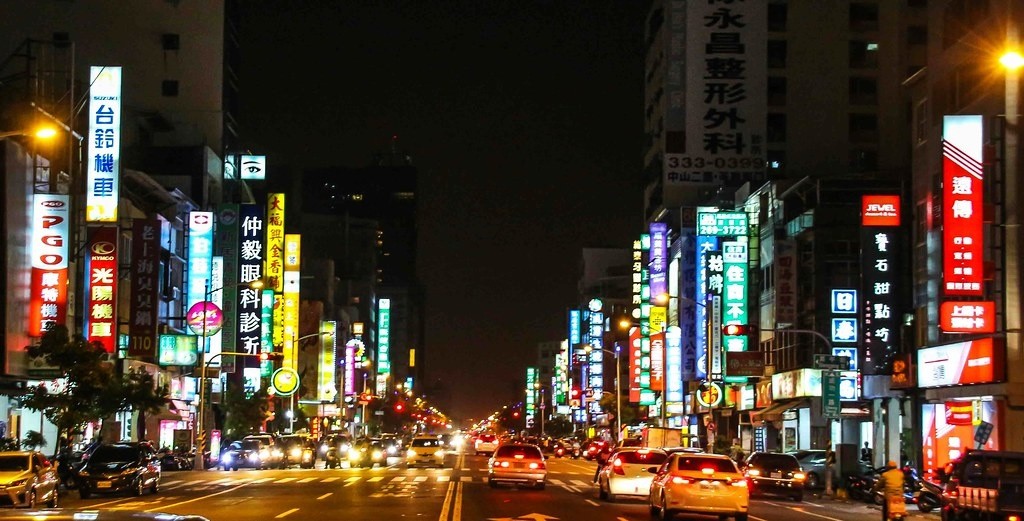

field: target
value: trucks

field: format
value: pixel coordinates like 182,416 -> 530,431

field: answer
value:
644,427 -> 685,449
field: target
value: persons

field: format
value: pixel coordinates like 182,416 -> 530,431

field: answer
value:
593,446 -> 610,483
872,460 -> 905,521
861,442 -> 872,461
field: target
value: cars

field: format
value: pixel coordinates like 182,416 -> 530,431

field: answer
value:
612,438 -> 643,449
489,443 -> 552,486
473,434 -> 500,456
52,438 -> 103,492
78,441 -> 170,496
740,451 -> 811,503
596,447 -> 670,503
649,453 -> 752,521
785,449 -> 867,489
0,452 -> 62,512
404,436 -> 448,469
435,430 -> 643,465
156,450 -> 216,470
1,505 -> 208,521
224,432 -> 402,470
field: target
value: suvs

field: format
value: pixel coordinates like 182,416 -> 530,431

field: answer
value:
939,449 -> 1024,521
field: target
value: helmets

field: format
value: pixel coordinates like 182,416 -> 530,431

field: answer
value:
886,460 -> 897,468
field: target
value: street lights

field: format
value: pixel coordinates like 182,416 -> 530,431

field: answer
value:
290,332 -> 335,436
655,292 -> 714,452
617,318 -> 668,450
583,344 -> 621,450
198,279 -> 264,474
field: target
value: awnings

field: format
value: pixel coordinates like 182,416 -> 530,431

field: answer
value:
752,400 -> 802,422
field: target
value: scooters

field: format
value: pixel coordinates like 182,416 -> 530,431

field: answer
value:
851,467 -> 944,512
876,491 -> 907,521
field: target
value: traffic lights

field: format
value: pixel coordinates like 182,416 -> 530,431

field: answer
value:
722,323 -> 761,340
260,351 -> 286,363
